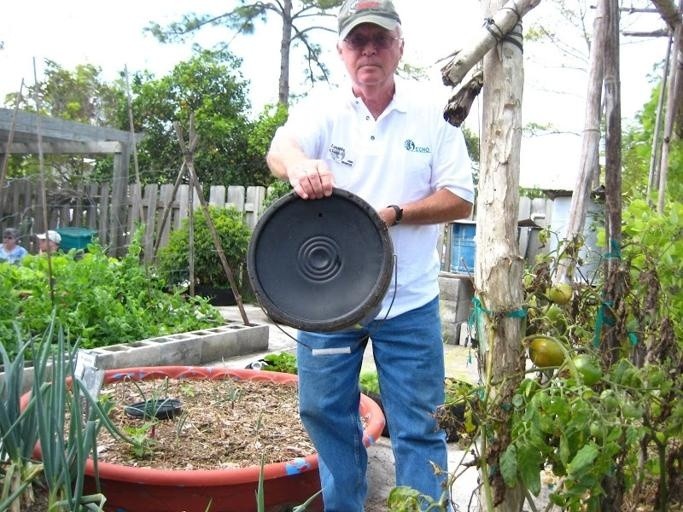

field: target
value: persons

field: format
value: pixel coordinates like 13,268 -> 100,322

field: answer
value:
0,228 -> 27,267
267,1 -> 475,512
36,231 -> 63,254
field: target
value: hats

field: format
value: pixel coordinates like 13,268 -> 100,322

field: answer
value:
337,0 -> 402,40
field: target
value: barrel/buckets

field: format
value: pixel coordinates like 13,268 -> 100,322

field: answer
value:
450,223 -> 475,275
247,187 -> 398,356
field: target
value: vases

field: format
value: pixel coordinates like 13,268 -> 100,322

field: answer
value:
11,364 -> 386,510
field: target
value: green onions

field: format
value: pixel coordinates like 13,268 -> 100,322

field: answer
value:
0,318 -> 139,512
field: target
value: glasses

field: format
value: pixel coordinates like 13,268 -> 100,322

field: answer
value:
343,30 -> 392,50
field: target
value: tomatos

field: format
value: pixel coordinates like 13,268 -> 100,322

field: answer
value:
547,281 -> 572,304
589,421 -> 608,438
601,390 -> 618,407
529,338 -> 564,366
651,372 -> 663,385
623,405 -> 641,420
572,354 -> 602,384
545,305 -> 563,320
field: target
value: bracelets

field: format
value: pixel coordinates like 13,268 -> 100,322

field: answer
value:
387,204 -> 403,227
